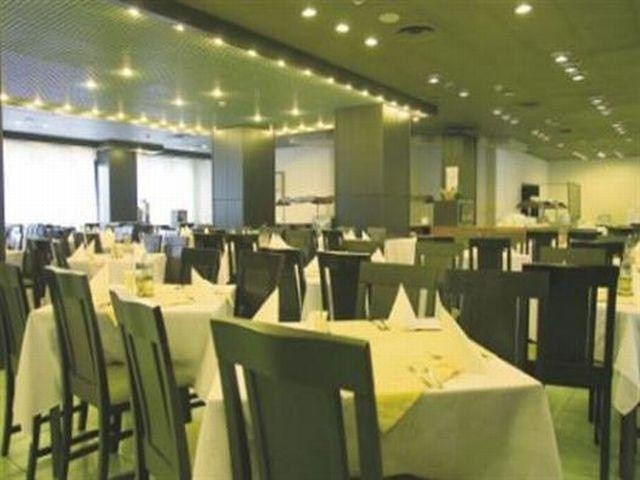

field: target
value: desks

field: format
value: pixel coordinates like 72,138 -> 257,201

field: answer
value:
609,315 -> 640,480
195,310 -> 566,480
11,280 -> 235,479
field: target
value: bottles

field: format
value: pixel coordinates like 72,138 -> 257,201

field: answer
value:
133,233 -> 156,298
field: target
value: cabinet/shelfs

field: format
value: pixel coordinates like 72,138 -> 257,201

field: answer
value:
435,200 -> 475,227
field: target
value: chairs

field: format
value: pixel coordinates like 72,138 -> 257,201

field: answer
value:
2,262 -> 89,479
203,315 -> 386,480
42,264 -> 194,479
514,261 -> 622,480
106,282 -> 193,478
0,218 -> 639,327
